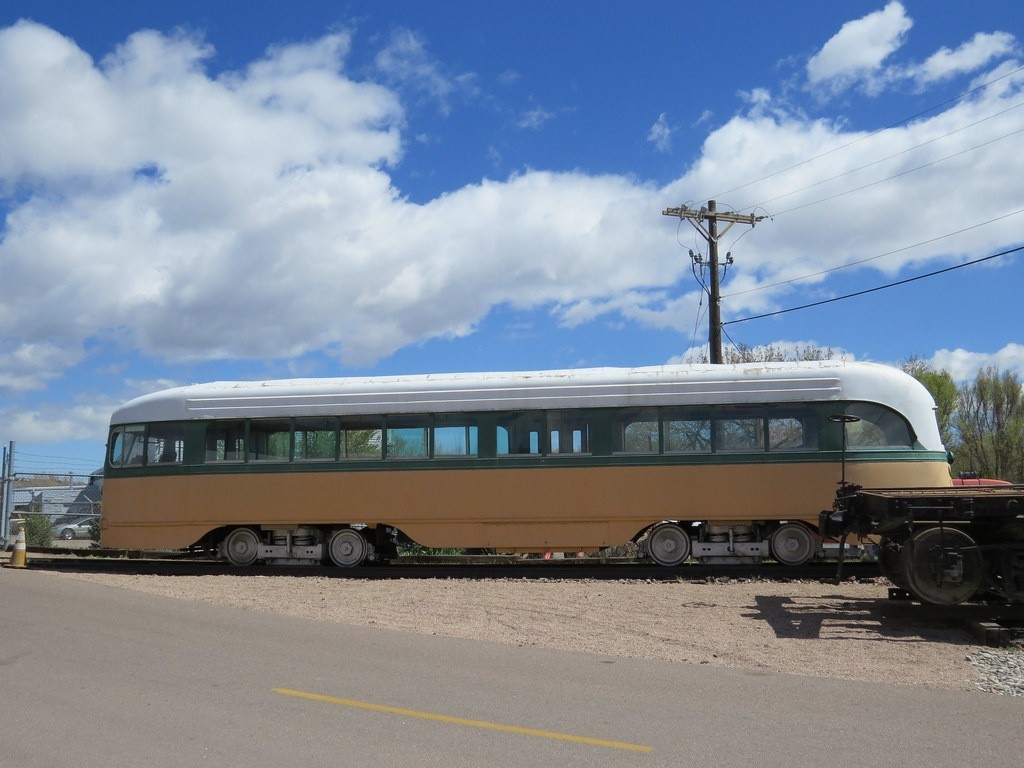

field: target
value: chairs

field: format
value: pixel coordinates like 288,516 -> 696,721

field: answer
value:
158,451 -> 177,463
131,455 -> 147,465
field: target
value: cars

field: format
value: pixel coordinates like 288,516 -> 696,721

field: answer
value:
51,515 -> 99,540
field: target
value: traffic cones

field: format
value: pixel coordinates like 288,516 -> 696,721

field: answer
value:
3,527 -> 32,570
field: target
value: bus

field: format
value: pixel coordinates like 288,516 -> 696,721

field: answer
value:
96,358 -> 959,572
